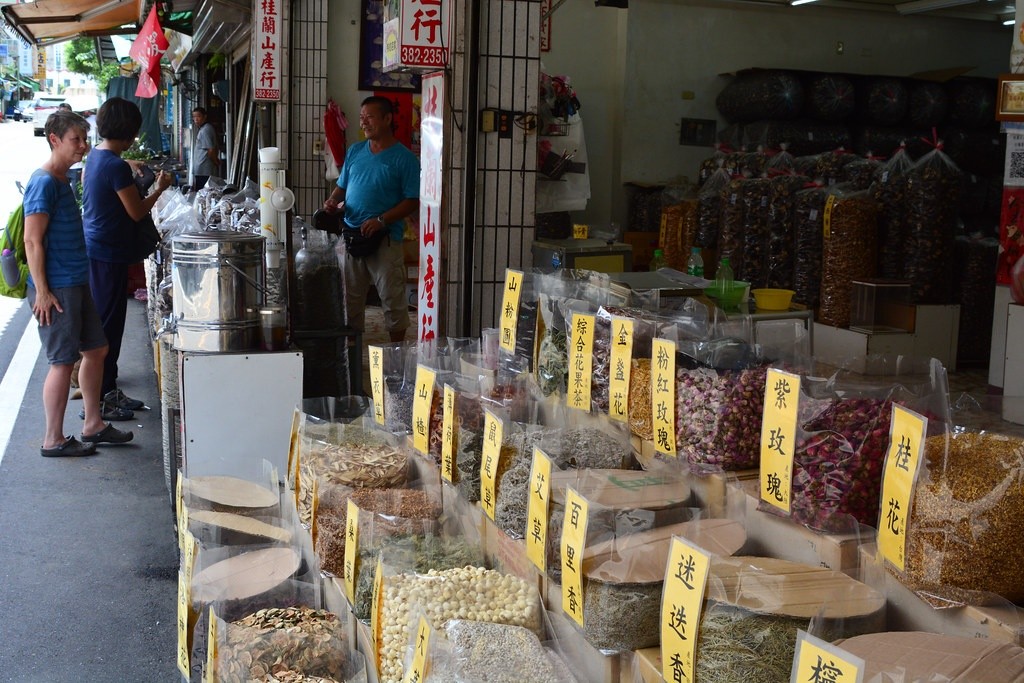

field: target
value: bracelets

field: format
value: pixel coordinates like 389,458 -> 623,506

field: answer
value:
155,190 -> 161,195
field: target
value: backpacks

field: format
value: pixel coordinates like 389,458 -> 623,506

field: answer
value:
0,203 -> 30,299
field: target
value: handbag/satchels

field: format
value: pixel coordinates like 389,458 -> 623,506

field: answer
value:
342,225 -> 392,259
128,186 -> 162,265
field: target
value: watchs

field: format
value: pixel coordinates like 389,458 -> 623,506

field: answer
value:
377,215 -> 385,228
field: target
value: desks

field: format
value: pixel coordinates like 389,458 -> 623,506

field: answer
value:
540,235 -> 631,274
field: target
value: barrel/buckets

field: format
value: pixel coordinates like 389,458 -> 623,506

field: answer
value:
170,232 -> 266,353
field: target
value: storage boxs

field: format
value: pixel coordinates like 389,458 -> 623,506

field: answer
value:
705,300 -> 814,368
295,372 -> 1024,683
849,280 -> 915,335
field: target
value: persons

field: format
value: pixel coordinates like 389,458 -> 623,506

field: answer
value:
60,96 -> 171,422
323,97 -> 420,383
192,107 -> 220,191
22,112 -> 133,457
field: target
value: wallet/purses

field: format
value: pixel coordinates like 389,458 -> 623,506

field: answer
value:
134,166 -> 155,199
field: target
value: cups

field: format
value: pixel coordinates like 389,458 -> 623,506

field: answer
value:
260,306 -> 286,350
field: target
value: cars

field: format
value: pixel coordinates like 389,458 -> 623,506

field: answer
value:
22,101 -> 37,123
13,100 -> 28,121
33,97 -> 66,136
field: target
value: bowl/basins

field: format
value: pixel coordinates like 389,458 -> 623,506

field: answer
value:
752,289 -> 796,309
704,281 -> 750,306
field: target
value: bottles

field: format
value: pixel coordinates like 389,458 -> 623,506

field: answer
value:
3,249 -> 17,285
687,248 -> 705,279
716,258 -> 735,316
649,249 -> 666,272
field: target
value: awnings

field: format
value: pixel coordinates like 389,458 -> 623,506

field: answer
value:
1,0 -> 146,50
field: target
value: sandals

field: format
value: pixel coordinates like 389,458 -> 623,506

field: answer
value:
40,434 -> 97,457
80,422 -> 134,444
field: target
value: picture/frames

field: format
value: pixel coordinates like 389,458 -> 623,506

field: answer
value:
357,0 -> 423,95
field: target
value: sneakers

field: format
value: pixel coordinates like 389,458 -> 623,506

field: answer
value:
100,389 -> 144,410
79,401 -> 134,420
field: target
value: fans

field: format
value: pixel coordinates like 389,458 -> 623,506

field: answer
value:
178,78 -> 201,102
